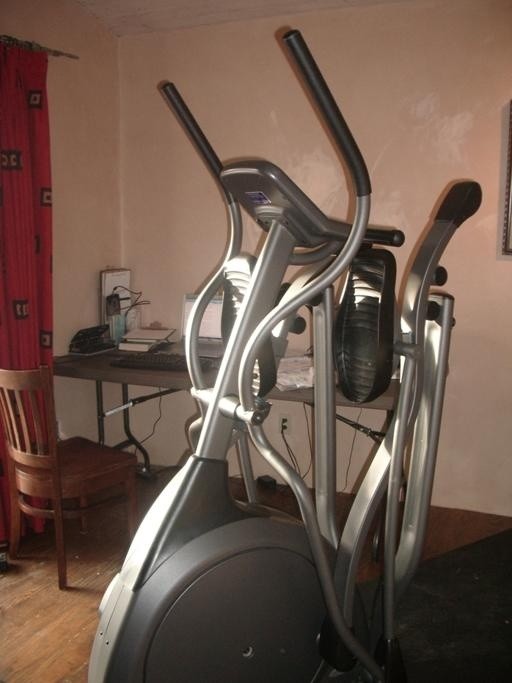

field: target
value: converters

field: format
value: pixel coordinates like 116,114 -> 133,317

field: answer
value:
258,475 -> 277,490
106,294 -> 120,315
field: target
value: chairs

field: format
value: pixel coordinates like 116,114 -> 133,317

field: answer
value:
1,362 -> 140,593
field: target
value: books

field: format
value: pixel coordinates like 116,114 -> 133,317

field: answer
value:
124,339 -> 158,343
118,341 -> 154,352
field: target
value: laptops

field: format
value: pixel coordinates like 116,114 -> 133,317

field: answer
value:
158,293 -> 224,358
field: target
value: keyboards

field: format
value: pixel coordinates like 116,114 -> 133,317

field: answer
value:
110,352 -> 213,372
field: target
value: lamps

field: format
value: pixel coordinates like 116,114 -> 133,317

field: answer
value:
106,283 -> 149,336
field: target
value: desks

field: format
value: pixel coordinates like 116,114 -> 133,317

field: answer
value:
51,341 -> 408,564
